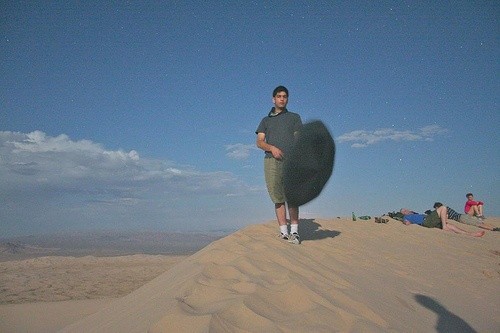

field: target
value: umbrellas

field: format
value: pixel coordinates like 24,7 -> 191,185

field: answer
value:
281,120 -> 336,208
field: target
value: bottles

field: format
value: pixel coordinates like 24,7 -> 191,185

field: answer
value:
358,215 -> 371,220
352,212 -> 355,221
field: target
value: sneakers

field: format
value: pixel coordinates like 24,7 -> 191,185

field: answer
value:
280,233 -> 289,240
289,232 -> 300,244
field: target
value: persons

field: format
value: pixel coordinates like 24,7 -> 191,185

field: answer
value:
433,202 -> 500,231
255,85 -> 310,241
464,193 -> 487,220
400,206 -> 485,237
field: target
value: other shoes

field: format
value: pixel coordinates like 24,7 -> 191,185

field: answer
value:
477,214 -> 485,219
